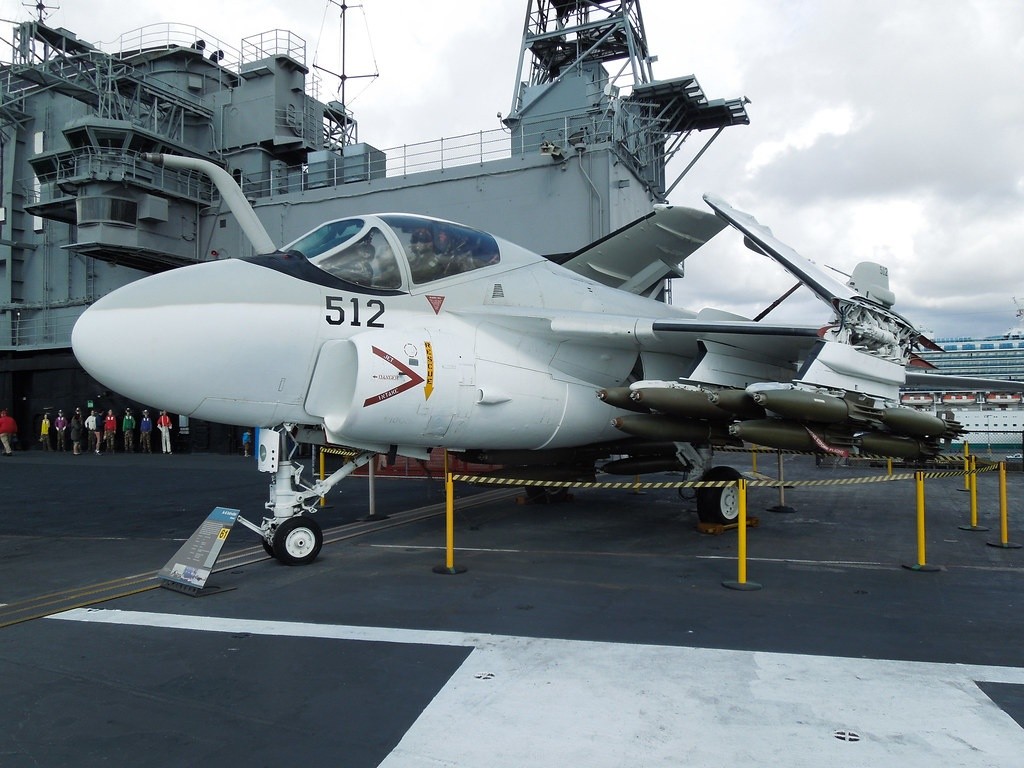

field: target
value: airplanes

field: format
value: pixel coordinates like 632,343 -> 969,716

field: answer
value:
70,192 -> 1024,568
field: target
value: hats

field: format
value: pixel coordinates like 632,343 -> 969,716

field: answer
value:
143,410 -> 147,413
58,410 -> 63,413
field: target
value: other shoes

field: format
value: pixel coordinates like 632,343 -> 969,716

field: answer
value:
162,451 -> 173,455
73,452 -> 82,456
1,451 -> 13,456
245,454 -> 251,457
94,450 -> 102,456
142,449 -> 153,454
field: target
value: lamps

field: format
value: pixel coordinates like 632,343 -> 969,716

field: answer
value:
604,83 -> 620,97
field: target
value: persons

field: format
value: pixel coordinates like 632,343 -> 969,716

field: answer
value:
40,407 -> 174,457
242,429 -> 253,457
406,226 -> 443,283
0,410 -> 17,457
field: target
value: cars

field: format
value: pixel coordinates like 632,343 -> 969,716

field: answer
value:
1006,453 -> 1023,461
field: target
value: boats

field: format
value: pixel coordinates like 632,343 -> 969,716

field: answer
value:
897,296 -> 1024,453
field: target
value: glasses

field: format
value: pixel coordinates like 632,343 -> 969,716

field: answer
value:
410,237 -> 432,244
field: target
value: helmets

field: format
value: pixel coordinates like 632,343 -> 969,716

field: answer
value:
410,227 -> 433,250
